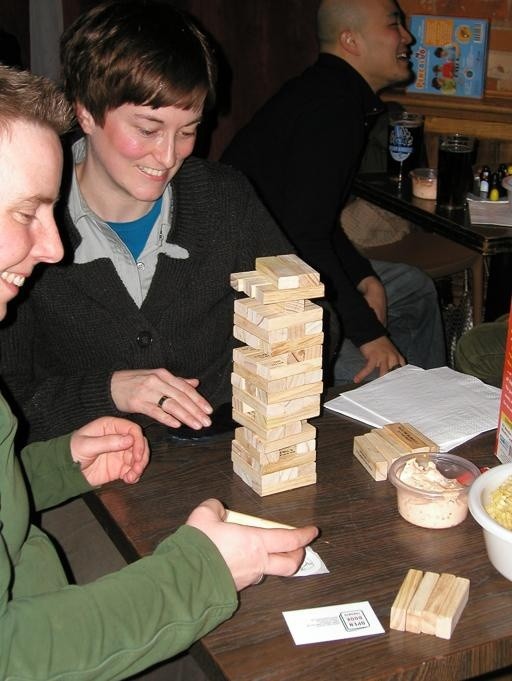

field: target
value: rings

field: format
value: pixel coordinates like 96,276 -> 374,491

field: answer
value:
157,393 -> 173,410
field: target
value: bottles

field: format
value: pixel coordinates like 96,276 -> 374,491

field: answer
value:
480,163 -> 512,201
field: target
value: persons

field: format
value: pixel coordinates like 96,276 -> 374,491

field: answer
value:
0,0 -> 351,446
210,0 -> 453,384
431,62 -> 458,79
433,41 -> 463,62
0,59 -> 327,679
448,305 -> 512,391
431,75 -> 456,95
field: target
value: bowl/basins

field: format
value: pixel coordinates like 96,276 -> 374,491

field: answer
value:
408,168 -> 439,199
468,461 -> 511,581
387,453 -> 478,530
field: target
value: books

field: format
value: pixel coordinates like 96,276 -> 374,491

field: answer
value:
403,14 -> 489,100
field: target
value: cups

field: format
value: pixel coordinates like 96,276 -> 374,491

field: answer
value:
386,111 -> 423,183
438,133 -> 472,210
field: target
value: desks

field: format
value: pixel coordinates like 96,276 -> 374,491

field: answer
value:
351,172 -> 512,322
82,384 -> 511,681
358,230 -> 484,328
